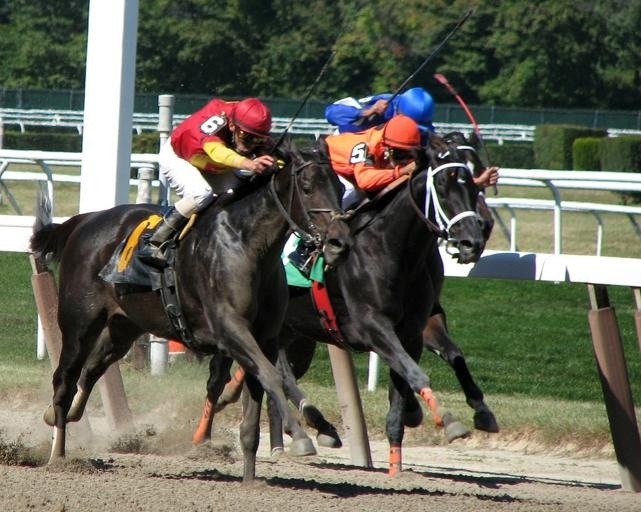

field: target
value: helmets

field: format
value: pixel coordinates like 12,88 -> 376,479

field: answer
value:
380,114 -> 421,150
399,87 -> 433,122
232,98 -> 271,138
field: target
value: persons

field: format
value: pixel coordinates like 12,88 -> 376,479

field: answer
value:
323,85 -> 436,150
286,111 -> 500,279
140,96 -> 276,265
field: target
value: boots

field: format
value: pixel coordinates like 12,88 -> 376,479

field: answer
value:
288,238 -> 317,267
139,208 -> 190,261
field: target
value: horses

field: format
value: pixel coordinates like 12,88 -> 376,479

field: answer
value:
30,134 -> 352,487
269,131 -> 494,461
191,131 -> 483,478
